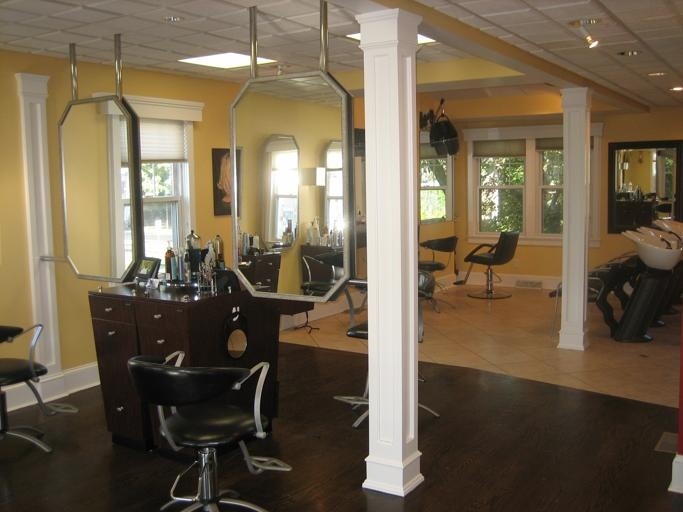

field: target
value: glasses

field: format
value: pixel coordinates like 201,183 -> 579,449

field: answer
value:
578,25 -> 601,51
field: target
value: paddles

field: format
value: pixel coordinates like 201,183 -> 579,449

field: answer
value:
1,340 -> 683,512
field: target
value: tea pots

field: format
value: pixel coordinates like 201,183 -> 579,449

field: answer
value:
231,68 -> 354,303
56,95 -> 146,285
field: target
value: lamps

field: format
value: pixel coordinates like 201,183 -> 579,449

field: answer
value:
298,248 -> 343,295
0,323 -> 79,453
333,229 -> 522,428
127,349 -> 292,512
606,137 -> 683,234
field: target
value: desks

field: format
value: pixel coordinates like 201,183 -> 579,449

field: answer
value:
165,190 -> 172,228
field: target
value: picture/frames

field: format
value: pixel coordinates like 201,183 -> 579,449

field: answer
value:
88,287 -> 279,454
239,252 -> 280,291
